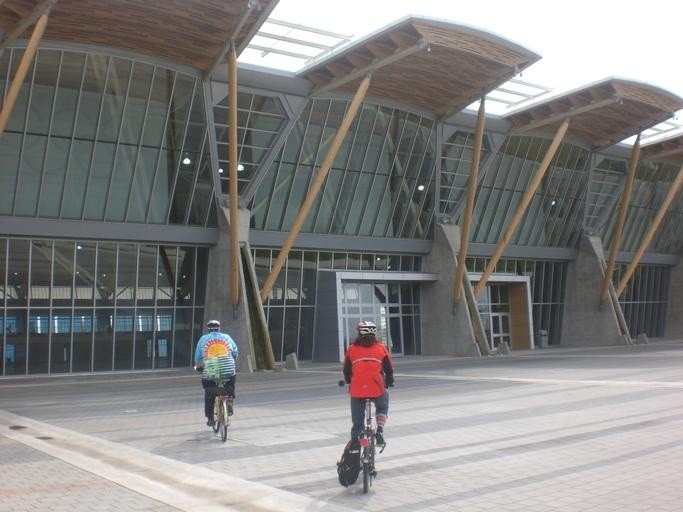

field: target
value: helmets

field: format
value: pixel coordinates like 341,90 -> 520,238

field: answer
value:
355,320 -> 377,337
206,319 -> 220,330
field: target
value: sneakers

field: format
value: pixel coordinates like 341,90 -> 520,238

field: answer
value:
225,398 -> 234,417
205,416 -> 216,427
374,426 -> 385,445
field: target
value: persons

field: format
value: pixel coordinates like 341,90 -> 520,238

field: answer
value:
191,319 -> 240,427
341,320 -> 395,447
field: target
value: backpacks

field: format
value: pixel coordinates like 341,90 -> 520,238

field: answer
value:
335,439 -> 361,488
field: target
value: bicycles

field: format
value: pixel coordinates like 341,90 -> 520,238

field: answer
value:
339,380 -> 386,493
196,367 -> 233,441
